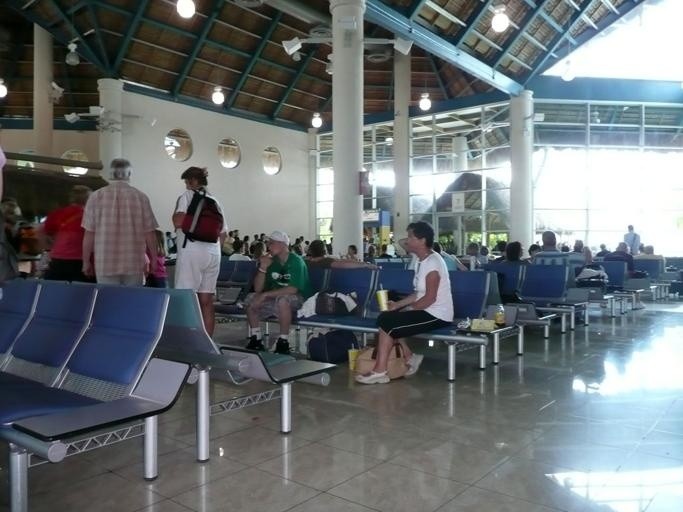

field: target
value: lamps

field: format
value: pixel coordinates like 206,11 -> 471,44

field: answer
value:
418,91 -> 431,113
0,78 -> 9,99
491,4 -> 510,34
281,34 -> 332,57
363,35 -> 414,56
120,112 -> 158,129
311,112 -> 324,130
63,108 -> 101,123
65,43 -> 80,68
211,84 -> 225,107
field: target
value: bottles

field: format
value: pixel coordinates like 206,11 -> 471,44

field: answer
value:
494,303 -> 505,327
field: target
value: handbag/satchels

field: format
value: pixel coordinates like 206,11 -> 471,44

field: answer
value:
308,330 -> 359,362
356,343 -> 409,379
316,290 -> 362,315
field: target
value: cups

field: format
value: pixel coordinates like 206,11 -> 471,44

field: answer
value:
376,290 -> 389,313
347,349 -> 358,370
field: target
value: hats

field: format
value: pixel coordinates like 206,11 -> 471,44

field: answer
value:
262,230 -> 289,246
182,167 -> 207,179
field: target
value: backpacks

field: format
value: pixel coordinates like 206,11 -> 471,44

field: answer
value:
182,188 -> 223,243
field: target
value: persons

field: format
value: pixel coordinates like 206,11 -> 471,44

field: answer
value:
79,156 -> 161,287
624,225 -> 640,256
173,166 -> 226,386
467,230 -> 656,323
36,183 -> 97,285
144,229 -> 334,355
355,221 -> 454,384
0,198 -> 31,282
363,236 -> 456,271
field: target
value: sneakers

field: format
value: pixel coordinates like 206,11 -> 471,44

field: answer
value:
274,338 -> 289,355
247,335 -> 264,351
355,368 -> 390,384
405,353 -> 424,375
